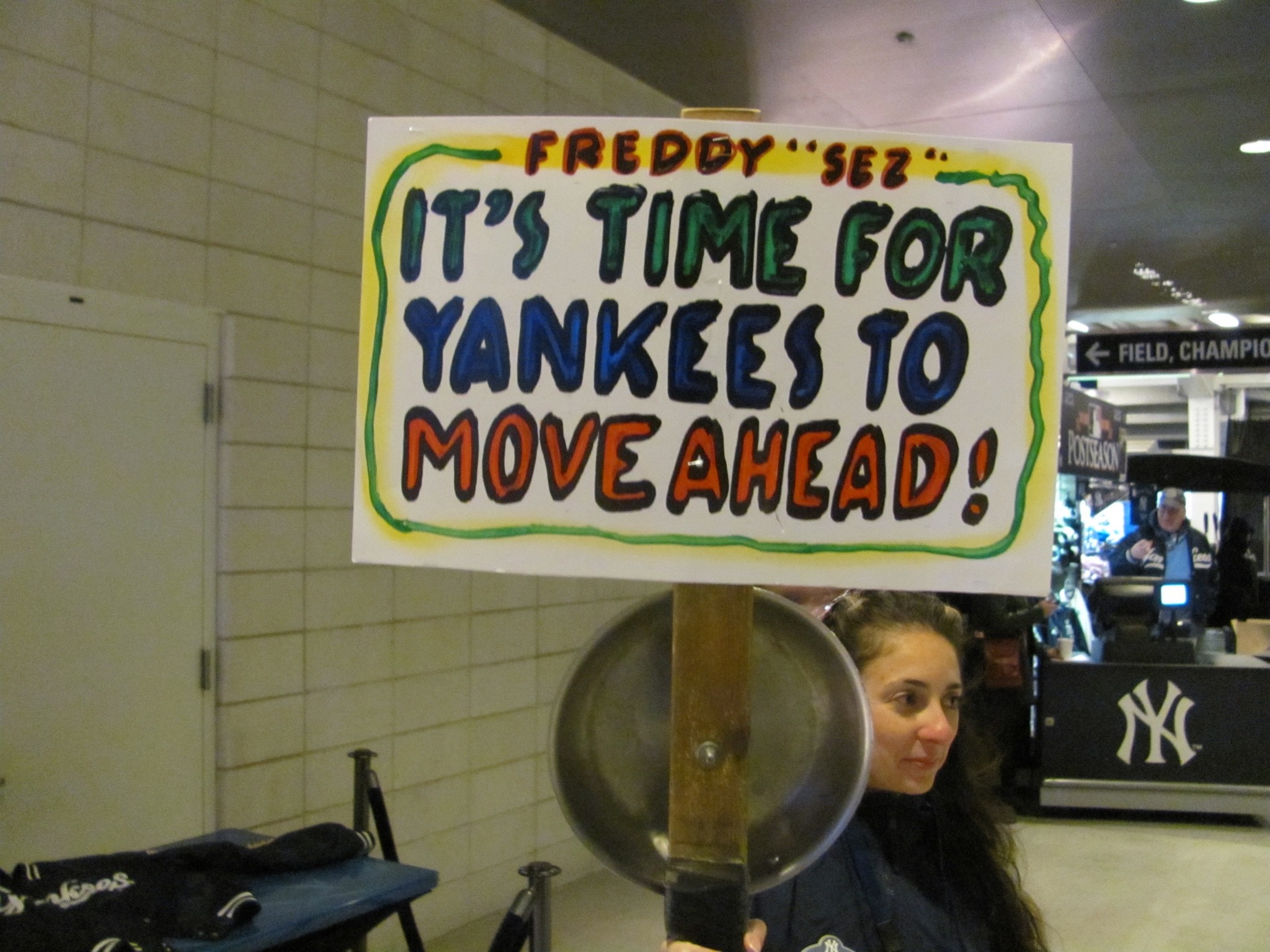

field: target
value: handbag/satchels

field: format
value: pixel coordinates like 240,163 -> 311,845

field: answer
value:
981,638 -> 1023,689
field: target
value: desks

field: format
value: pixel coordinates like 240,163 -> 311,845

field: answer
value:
0,828 -> 439,952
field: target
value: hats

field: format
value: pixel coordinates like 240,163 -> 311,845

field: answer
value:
1157,487 -> 1186,510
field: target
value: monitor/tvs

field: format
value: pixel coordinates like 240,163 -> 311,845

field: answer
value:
1091,575 -> 1164,627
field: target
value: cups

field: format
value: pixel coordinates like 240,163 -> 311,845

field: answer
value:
1057,638 -> 1074,662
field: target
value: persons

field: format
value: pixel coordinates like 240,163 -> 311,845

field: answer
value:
657,588 -> 1059,952
1210,513 -> 1260,623
1108,486 -> 1213,631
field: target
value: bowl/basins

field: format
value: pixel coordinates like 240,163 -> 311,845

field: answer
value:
550,587 -> 874,898
